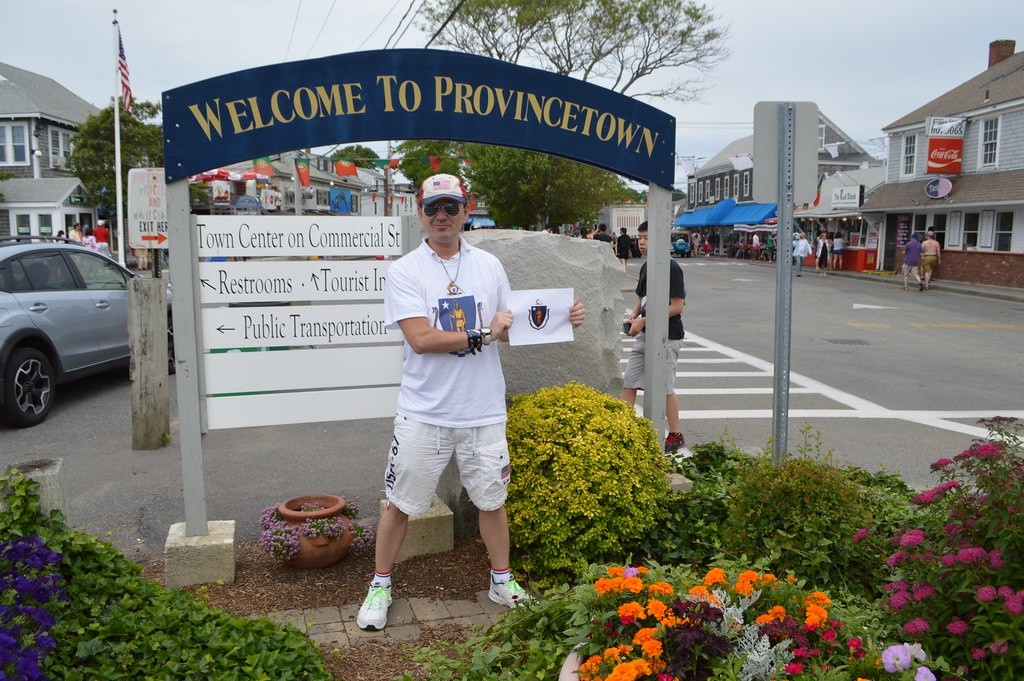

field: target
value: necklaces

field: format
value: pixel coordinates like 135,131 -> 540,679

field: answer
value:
441,250 -> 461,293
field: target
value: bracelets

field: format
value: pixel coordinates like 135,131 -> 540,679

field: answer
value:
466,327 -> 492,349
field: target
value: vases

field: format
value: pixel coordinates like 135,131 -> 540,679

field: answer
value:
558,634 -> 594,681
276,494 -> 354,570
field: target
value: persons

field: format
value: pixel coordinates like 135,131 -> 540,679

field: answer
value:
543,224 -> 551,232
56,220 -> 109,257
793,232 -> 844,276
357,173 -> 583,631
689,230 -> 716,258
135,248 -> 149,270
565,223 -> 613,246
617,228 -> 631,274
902,227 -> 941,291
768,231 -> 776,263
624,220 -> 686,453
753,232 -> 760,260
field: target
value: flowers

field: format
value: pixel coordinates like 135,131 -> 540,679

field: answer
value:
257,494 -> 376,568
563,564 -> 936,681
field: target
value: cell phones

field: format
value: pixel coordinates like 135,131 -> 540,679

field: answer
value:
622,322 -> 632,333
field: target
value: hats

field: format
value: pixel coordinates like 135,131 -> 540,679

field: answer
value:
97,220 -> 107,226
924,231 -> 933,236
912,233 -> 917,238
420,174 -> 467,205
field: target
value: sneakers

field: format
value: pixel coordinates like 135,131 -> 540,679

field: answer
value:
665,432 -> 685,453
488,575 -> 540,609
356,582 -> 392,629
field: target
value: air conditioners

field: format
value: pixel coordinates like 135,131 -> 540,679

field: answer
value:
53,155 -> 66,166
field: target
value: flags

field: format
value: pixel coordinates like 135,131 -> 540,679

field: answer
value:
118,31 -> 132,112
253,155 -> 475,188
469,191 -> 479,210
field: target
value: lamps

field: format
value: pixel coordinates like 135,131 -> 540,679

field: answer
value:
329,176 -> 334,186
34,124 -> 42,138
912,197 -> 919,206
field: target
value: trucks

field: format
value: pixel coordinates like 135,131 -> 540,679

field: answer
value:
597,203 -> 647,258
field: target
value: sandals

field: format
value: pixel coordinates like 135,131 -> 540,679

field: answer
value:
919,283 -> 923,291
901,287 -> 908,290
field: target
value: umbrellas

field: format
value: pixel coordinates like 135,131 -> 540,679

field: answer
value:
196,169 -> 269,181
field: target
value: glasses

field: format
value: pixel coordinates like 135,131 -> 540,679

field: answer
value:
424,201 -> 460,217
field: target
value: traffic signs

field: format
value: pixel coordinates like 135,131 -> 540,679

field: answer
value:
201,303 -> 405,350
198,261 -> 397,304
125,168 -> 169,250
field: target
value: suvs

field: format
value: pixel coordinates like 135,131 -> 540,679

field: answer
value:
0,232 -> 174,429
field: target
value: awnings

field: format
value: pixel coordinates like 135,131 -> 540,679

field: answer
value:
793,209 -> 880,236
672,199 -> 776,226
